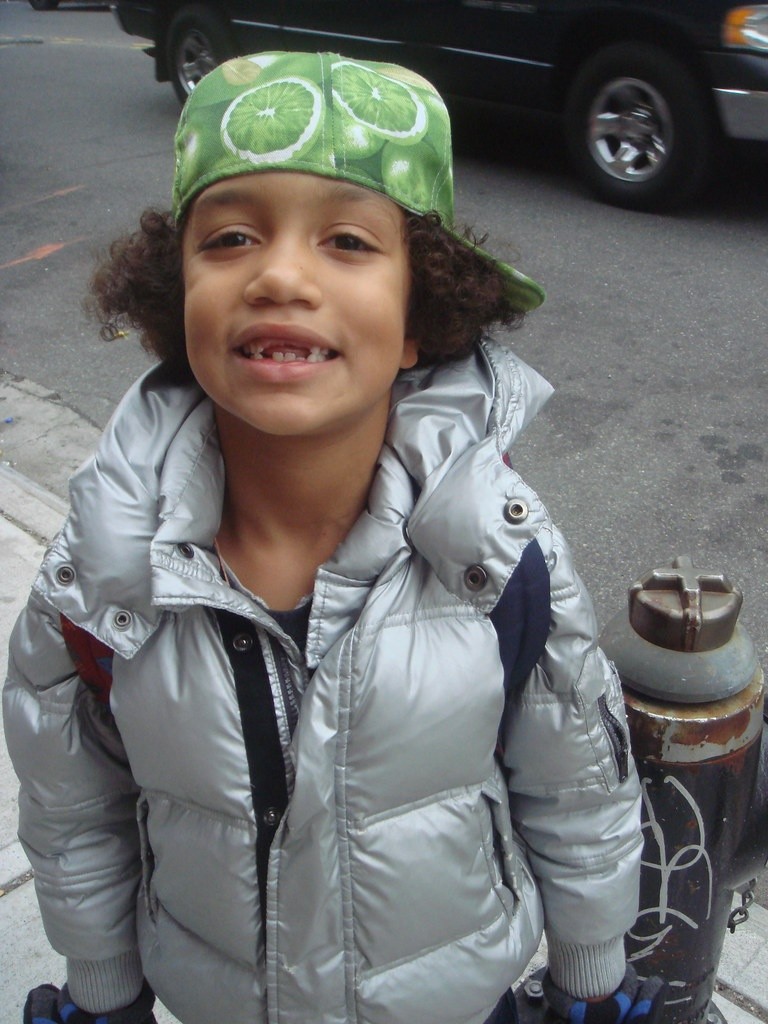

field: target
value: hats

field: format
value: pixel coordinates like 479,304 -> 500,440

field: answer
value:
171,48 -> 546,313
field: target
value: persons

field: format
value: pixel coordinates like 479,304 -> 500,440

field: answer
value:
0,44 -> 653,1024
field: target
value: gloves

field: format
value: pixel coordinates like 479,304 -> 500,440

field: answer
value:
23,975 -> 159,1024
541,962 -> 665,1024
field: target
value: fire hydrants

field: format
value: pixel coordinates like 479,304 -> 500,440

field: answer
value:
597,554 -> 768,1015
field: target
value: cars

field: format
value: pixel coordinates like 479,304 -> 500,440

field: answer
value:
107,3 -> 768,206
